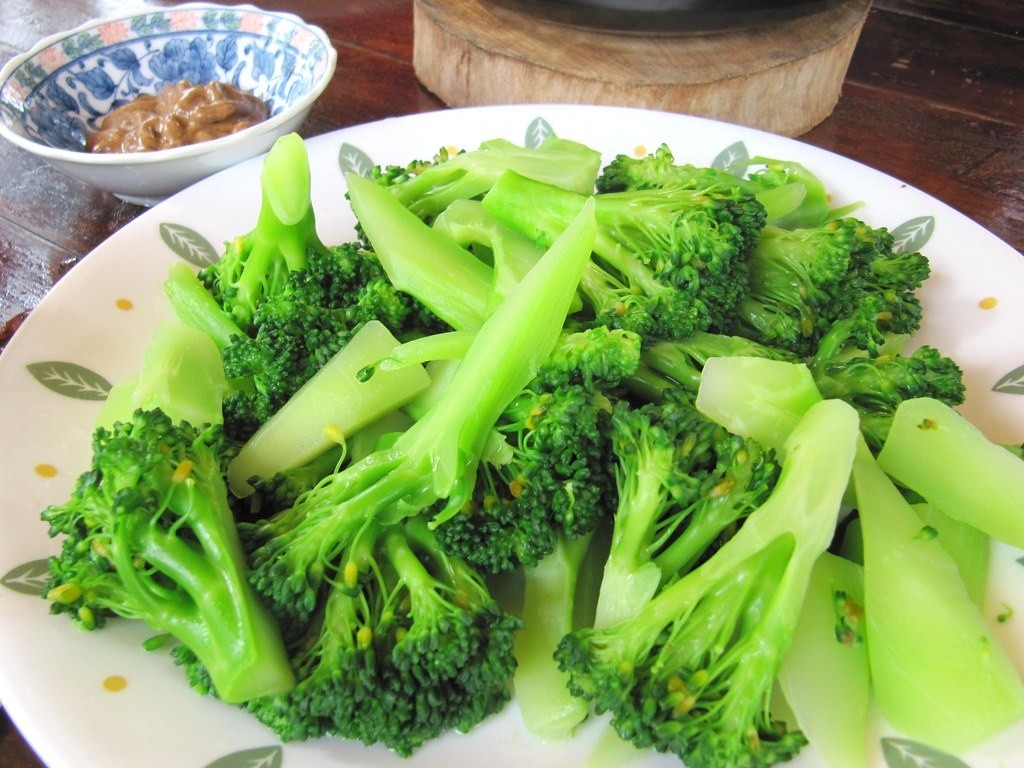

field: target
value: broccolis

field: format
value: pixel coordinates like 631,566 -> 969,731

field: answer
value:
42,131 -> 1024,767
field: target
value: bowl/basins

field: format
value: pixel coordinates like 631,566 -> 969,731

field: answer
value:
0,2 -> 338,206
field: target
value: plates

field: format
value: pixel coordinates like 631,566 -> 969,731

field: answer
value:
0,103 -> 1024,768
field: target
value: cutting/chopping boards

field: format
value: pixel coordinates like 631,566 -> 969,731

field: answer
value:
412,0 -> 873,139
